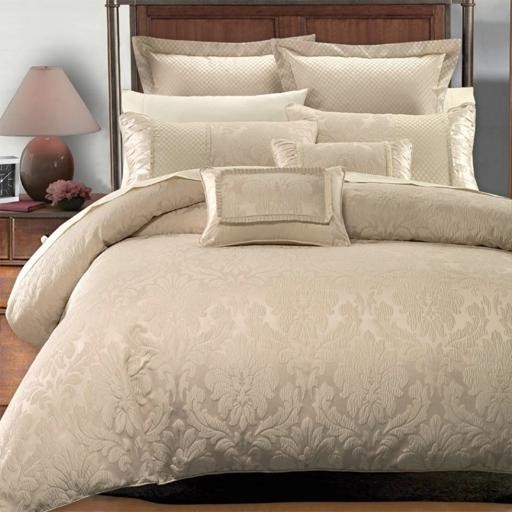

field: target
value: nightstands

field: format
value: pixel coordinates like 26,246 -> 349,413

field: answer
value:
0,194 -> 107,314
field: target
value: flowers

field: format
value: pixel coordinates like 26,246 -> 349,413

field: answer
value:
43,180 -> 95,202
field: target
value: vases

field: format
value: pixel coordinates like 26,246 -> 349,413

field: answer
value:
55,196 -> 86,211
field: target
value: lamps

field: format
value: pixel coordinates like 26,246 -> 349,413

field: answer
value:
0,65 -> 103,202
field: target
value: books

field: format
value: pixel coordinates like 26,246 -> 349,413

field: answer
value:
0,199 -> 46,213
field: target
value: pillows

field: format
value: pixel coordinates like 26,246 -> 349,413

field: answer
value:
270,37 -> 466,114
115,111 -> 320,187
120,89 -> 310,122
269,138 -> 417,182
128,32 -> 316,95
283,102 -> 481,192
198,166 -> 353,251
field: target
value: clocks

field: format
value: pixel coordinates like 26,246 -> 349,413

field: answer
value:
0,155 -> 21,204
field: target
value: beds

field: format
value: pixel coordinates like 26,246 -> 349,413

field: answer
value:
0,1 -> 509,512
